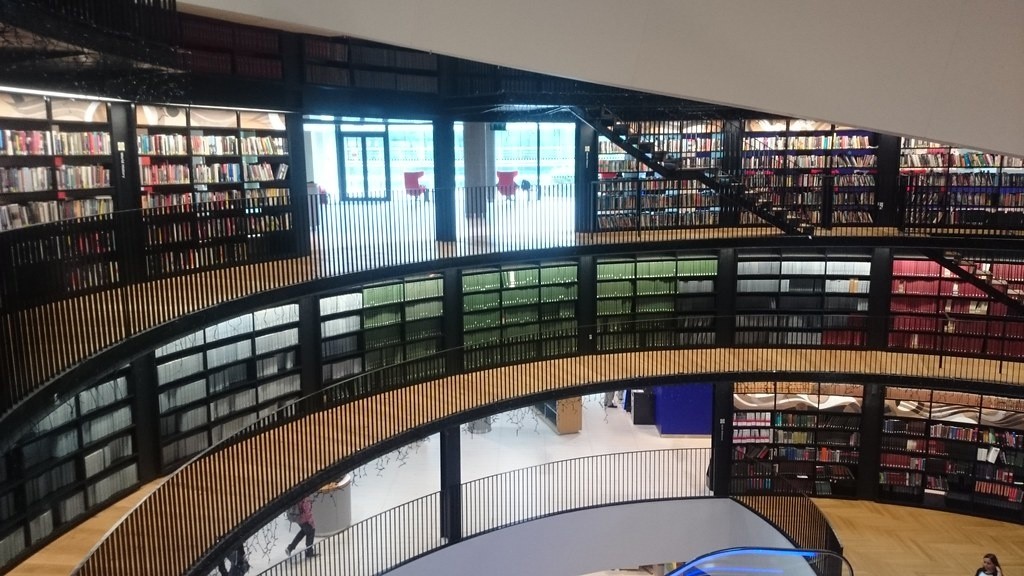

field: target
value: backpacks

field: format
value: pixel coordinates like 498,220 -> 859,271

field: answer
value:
286,503 -> 301,522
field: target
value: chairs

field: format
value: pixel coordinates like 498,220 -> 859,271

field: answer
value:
404,170 -> 428,206
495,169 -> 520,202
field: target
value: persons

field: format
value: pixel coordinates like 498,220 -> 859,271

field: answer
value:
975,554 -> 1003,576
605,391 -> 617,408
286,498 -> 321,558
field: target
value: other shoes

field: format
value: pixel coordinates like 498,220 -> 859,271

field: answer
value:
306,554 -> 319,559
285,548 -> 292,557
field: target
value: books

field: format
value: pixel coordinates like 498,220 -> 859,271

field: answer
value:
736,262 -> 871,345
739,136 -> 876,223
0,377 -> 138,569
138,134 -> 294,274
597,260 -> 717,350
729,413 -> 1024,511
898,135 -> 1024,226
0,129 -> 120,291
597,138 -> 719,225
156,305 -> 301,463
889,261 -> 1024,358
320,278 -> 446,402
462,266 -> 579,369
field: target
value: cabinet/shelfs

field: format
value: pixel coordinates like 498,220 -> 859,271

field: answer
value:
573,123 -> 899,227
177,14 -> 631,99
899,133 -> 1024,229
0,249 -> 1024,575
0,116 -> 311,312
